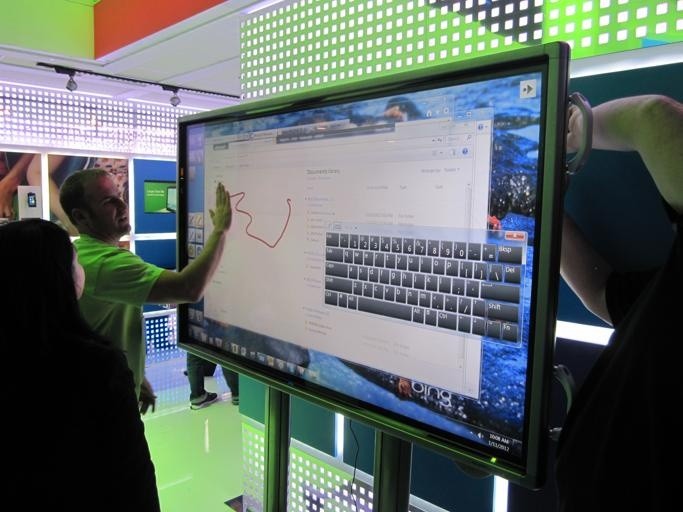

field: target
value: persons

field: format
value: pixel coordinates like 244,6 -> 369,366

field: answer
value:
0,152 -> 34,236
0,217 -> 160,510
57,167 -> 231,422
24,151 -> 130,236
553,91 -> 681,509
185,348 -> 238,409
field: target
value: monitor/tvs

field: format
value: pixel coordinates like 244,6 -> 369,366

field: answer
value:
176,41 -> 572,493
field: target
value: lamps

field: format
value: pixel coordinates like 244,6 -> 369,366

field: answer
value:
36,62 -> 180,107
